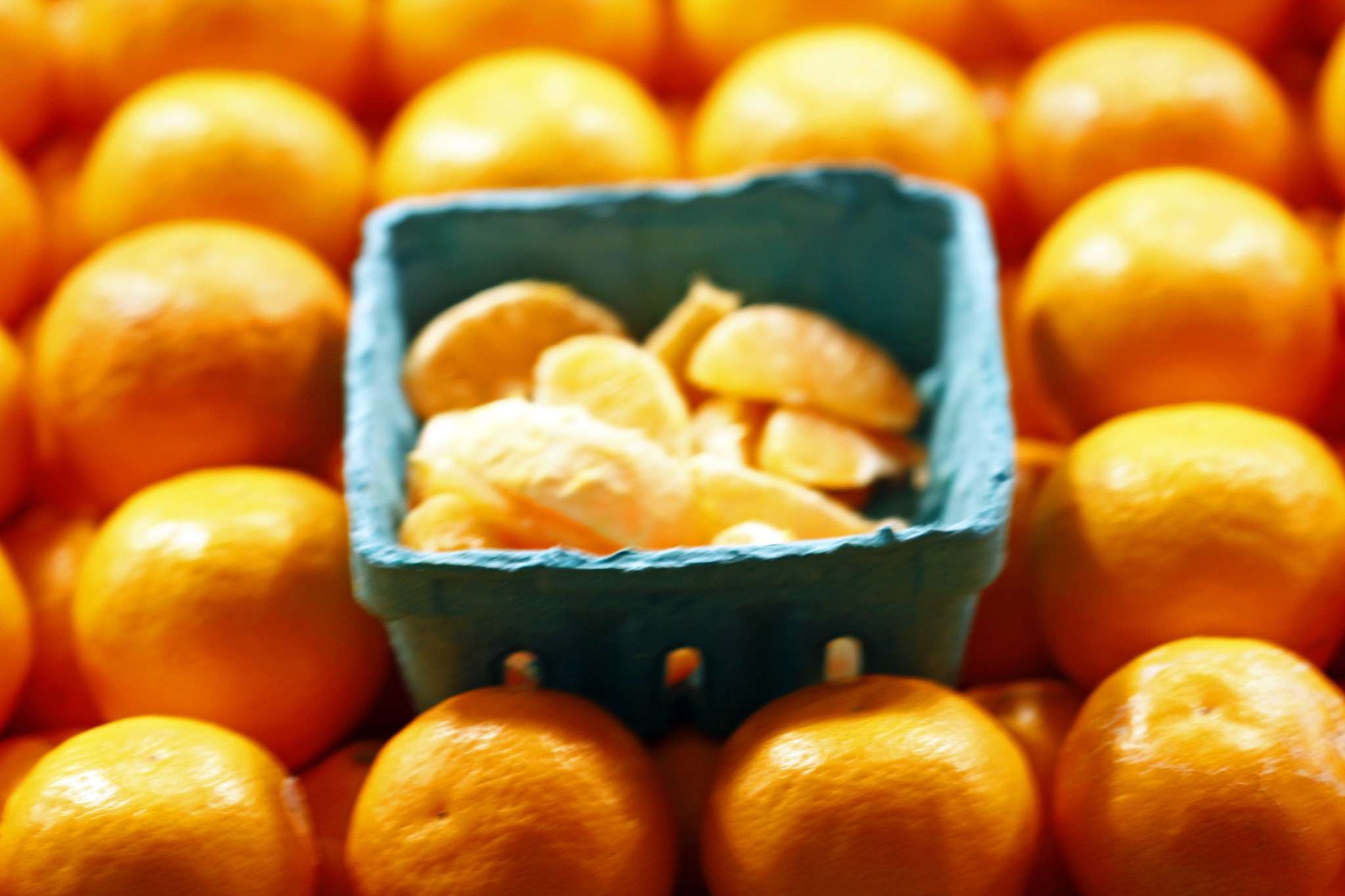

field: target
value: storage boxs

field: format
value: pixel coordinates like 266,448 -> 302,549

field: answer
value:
344,168 -> 1015,708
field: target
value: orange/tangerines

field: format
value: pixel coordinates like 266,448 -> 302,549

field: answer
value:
0,0 -> 1345,896
